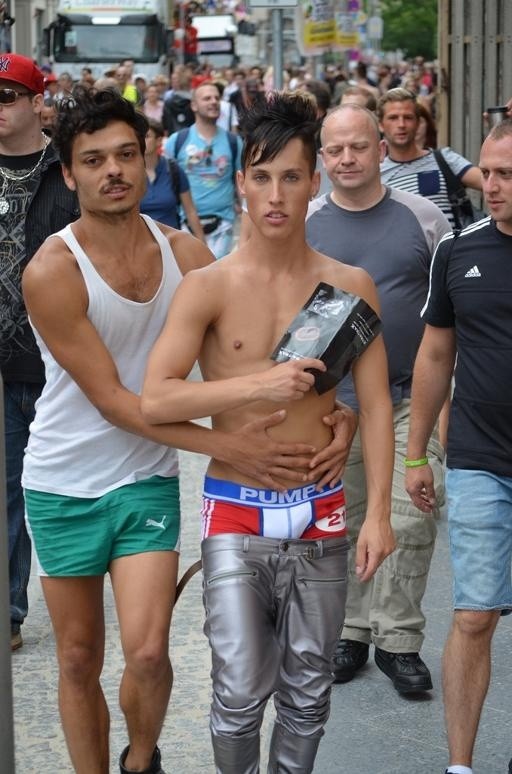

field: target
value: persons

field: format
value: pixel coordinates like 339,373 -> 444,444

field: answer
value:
301,106 -> 445,692
22,97 -> 217,769
404,114 -> 512,773
137,94 -> 398,773
0,43 -> 511,648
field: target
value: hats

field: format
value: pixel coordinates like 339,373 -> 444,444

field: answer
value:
45,73 -> 58,84
0,52 -> 44,96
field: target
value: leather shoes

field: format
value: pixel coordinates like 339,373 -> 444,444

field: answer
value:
330,638 -> 370,682
374,645 -> 432,694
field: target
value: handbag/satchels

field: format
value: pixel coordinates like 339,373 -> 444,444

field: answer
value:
453,194 -> 487,237
184,212 -> 220,235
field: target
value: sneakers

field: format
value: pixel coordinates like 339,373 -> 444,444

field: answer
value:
120,743 -> 165,773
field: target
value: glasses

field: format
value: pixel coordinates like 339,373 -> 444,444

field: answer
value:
204,143 -> 217,167
1,88 -> 33,105
